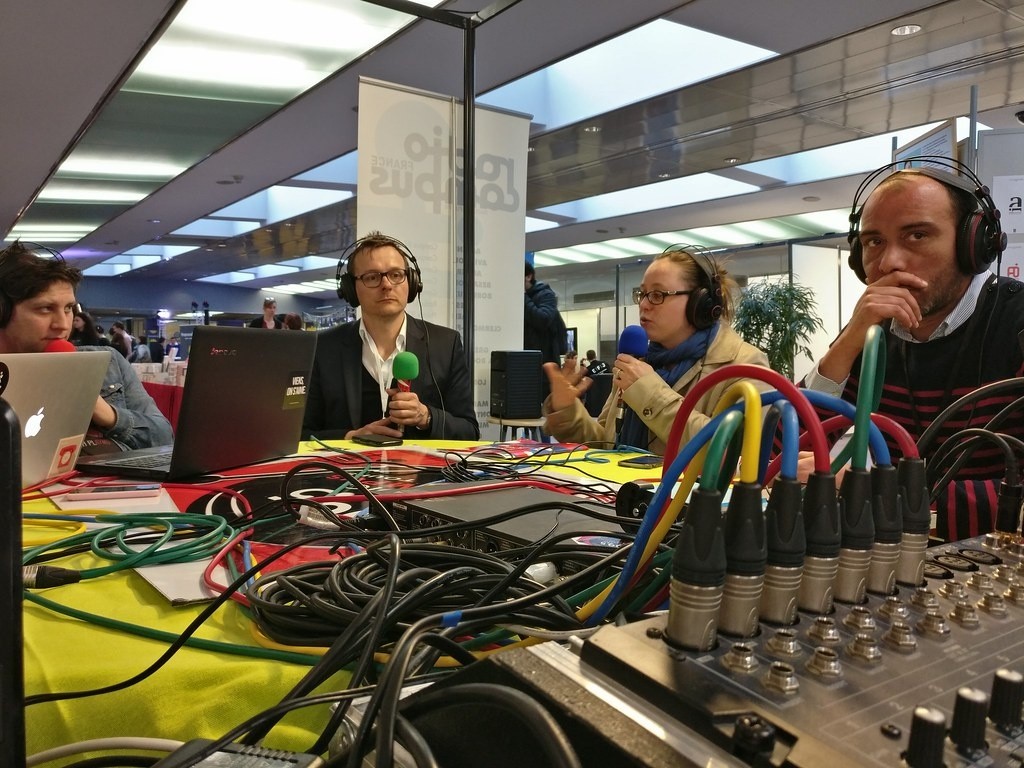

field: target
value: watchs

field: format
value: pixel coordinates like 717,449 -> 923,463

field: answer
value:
415,411 -> 433,431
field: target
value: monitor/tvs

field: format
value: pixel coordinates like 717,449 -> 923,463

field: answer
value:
559,327 -> 577,360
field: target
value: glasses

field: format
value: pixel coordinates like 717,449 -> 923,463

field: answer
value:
354,269 -> 408,288
265,297 -> 275,303
632,290 -> 692,305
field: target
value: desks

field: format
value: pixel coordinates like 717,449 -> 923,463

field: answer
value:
142,381 -> 184,435
24,436 -> 740,768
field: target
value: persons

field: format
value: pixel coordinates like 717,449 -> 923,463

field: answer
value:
563,348 -> 612,378
770,165 -> 1024,543
299,231 -> 480,441
524,262 -> 568,395
249,297 -> 303,331
542,250 -> 776,471
0,238 -> 174,459
69,312 -> 180,363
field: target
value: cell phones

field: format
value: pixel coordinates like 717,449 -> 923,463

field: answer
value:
67,484 -> 162,501
618,455 -> 665,469
351,434 -> 403,446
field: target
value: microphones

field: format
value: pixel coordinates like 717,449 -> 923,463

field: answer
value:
615,325 -> 648,434
392,351 -> 419,432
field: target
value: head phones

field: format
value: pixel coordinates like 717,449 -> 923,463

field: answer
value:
0,242 -> 82,328
336,234 -> 423,308
662,243 -> 724,330
847,156 -> 1007,285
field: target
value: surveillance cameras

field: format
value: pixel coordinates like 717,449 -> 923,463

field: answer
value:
1014,111 -> 1024,124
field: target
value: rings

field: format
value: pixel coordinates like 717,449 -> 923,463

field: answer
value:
615,369 -> 621,378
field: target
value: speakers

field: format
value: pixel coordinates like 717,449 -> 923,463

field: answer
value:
490,350 -> 543,419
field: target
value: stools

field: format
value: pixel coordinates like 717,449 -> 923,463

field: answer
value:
486,417 -> 547,441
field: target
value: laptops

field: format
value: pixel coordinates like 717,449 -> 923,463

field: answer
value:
75,326 -> 318,479
0,351 -> 111,490
